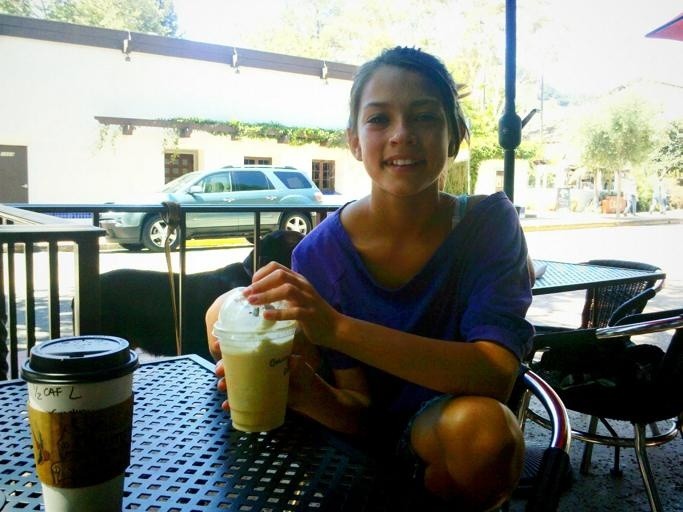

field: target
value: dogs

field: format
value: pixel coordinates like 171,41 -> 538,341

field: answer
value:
71,230 -> 305,365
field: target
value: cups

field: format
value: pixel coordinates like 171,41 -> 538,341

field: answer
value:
19,335 -> 138,512
212,289 -> 297,434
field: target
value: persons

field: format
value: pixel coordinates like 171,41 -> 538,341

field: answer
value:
215,43 -> 536,512
568,160 -> 674,218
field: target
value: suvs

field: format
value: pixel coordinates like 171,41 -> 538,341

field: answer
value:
99,166 -> 326,252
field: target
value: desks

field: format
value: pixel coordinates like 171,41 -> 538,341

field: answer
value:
442,257 -> 665,295
0,354 -> 444,512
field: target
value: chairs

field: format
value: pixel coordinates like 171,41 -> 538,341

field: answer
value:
525,305 -> 678,511
576,256 -> 668,332
500,368 -> 573,510
